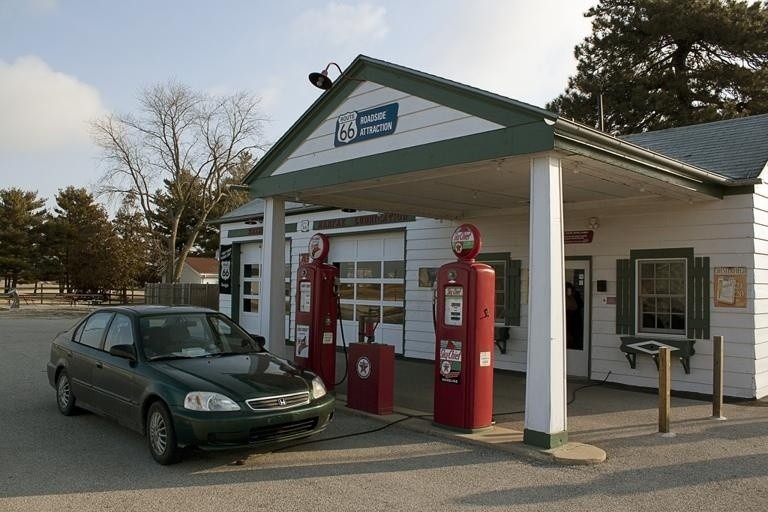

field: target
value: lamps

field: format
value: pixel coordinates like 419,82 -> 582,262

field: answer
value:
308,61 -> 365,90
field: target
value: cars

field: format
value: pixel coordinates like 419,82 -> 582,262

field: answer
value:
45,303 -> 335,467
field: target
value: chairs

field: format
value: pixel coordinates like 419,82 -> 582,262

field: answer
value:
147,326 -> 207,353
